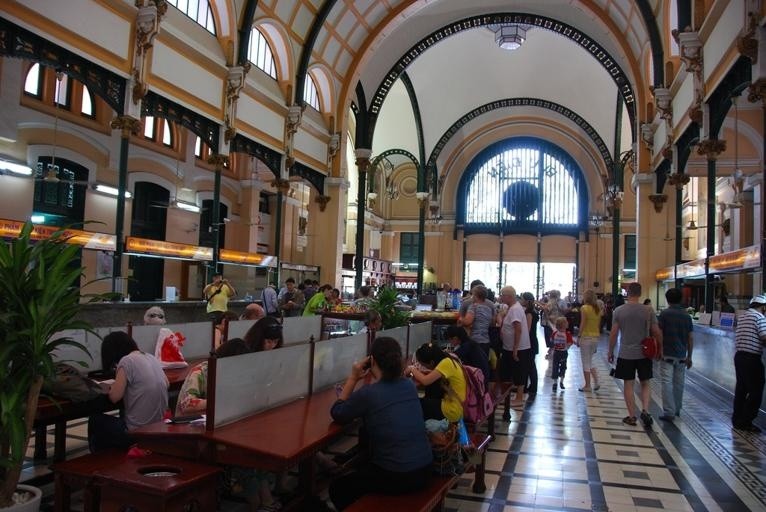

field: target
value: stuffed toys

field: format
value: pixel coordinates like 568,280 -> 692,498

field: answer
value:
143,305 -> 168,327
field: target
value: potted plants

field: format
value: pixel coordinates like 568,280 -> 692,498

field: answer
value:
0,214 -> 109,512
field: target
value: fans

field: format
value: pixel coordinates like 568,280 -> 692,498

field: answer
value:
654,204 -> 696,243
222,154 -> 271,227
30,70 -> 94,187
672,176 -> 723,230
292,179 -> 317,237
148,122 -> 194,213
714,100 -> 762,209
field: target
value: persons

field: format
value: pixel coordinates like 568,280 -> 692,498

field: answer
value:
174,338 -> 284,512
328,337 -> 435,511
86,330 -> 171,455
694,305 -> 704,318
607,282 -> 663,425
243,278 -> 342,320
214,310 -> 239,350
656,288 -> 696,423
243,315 -> 348,501
454,279 -> 625,395
202,272 -> 236,321
720,296 -> 735,314
358,286 -> 370,298
731,295 -> 766,433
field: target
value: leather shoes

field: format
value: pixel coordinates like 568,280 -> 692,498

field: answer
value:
658,415 -> 672,422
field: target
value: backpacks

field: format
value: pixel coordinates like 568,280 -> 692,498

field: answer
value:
49,362 -> 101,403
445,352 -> 494,424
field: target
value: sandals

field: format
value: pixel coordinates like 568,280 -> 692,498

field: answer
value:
640,409 -> 653,425
622,416 -> 637,426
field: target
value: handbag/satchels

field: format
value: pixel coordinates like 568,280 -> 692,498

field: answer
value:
428,425 -> 466,475
641,338 -> 660,358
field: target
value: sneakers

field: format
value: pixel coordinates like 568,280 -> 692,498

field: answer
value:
560,383 -> 565,389
553,383 -> 557,390
578,388 -> 592,392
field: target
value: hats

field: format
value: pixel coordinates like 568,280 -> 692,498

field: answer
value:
750,295 -> 766,304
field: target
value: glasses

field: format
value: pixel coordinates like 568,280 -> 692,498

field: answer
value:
147,312 -> 164,319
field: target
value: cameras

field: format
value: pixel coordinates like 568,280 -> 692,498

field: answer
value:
220,280 -> 223,284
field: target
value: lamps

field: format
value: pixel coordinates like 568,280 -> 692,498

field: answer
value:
493,25 -> 529,52
0,157 -> 36,178
416,191 -> 432,209
168,198 -> 204,216
93,181 -> 133,202
